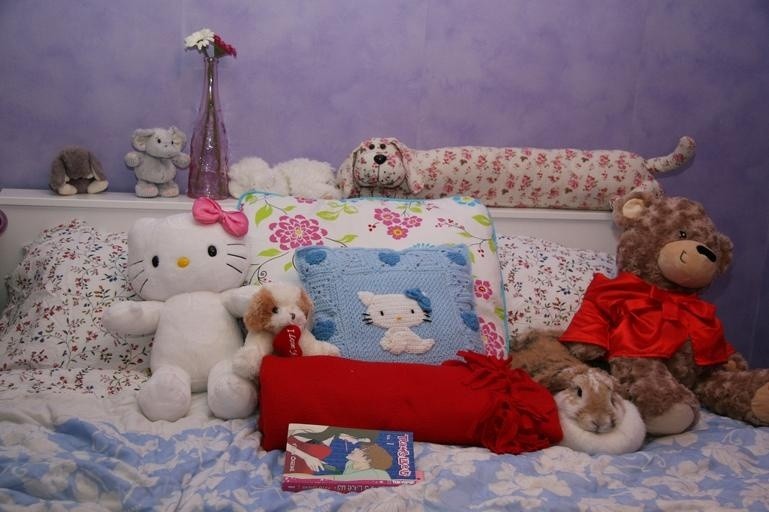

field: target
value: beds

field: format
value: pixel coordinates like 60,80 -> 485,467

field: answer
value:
0,194 -> 769,512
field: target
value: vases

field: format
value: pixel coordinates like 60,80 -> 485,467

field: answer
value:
190,55 -> 229,200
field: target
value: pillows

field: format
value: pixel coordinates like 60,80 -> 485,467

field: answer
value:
0,221 -> 154,398
496,236 -> 616,339
238,193 -> 510,362
259,353 -> 563,456
297,243 -> 484,362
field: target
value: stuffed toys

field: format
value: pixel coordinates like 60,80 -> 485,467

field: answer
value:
337,137 -> 696,209
507,329 -> 646,455
48,148 -> 109,198
228,156 -> 341,200
101,197 -> 262,422
558,190 -> 769,436
232,284 -> 341,389
125,125 -> 191,197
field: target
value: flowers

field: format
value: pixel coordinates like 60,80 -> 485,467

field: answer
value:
182,28 -> 237,195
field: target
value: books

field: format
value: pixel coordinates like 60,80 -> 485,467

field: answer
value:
281,422 -> 425,493
